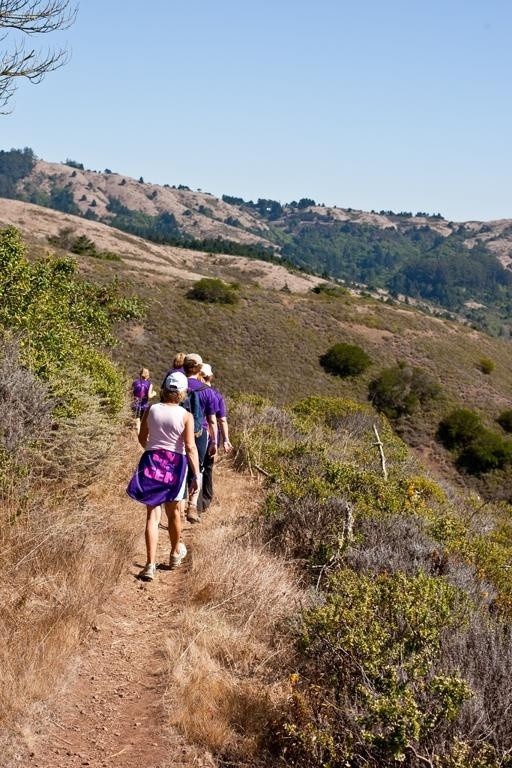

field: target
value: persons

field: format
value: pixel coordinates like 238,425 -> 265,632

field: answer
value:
126,372 -> 200,581
160,352 -> 233,523
132,368 -> 157,436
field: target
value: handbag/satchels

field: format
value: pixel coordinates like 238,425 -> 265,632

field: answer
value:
130,402 -> 142,418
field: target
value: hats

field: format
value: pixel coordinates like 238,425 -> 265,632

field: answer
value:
166,372 -> 188,392
200,363 -> 213,378
184,353 -> 202,366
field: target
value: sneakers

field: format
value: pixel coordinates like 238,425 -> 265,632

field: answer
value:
140,564 -> 154,580
188,508 -> 201,523
170,542 -> 187,570
